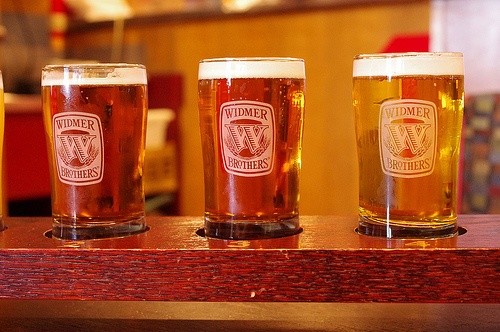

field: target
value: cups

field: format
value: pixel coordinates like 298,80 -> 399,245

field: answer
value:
194,57 -> 307,239
354,51 -> 464,237
38,61 -> 149,239
